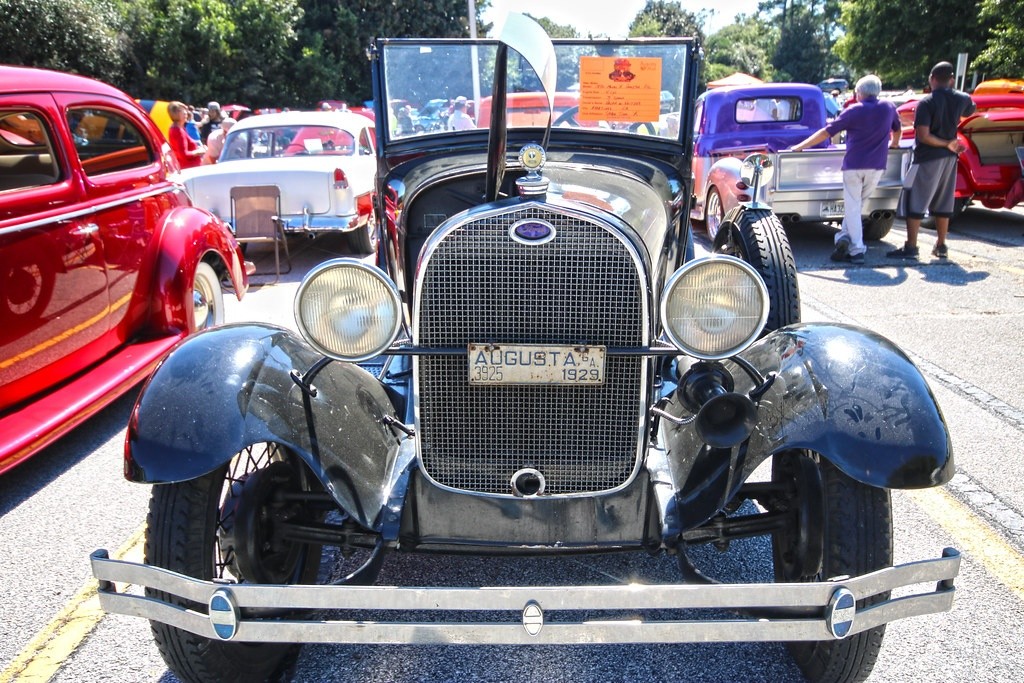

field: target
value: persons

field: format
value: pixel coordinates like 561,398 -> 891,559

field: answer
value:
831,88 -> 857,109
887,62 -> 975,258
396,95 -> 477,136
790,74 -> 902,263
903,83 -> 931,96
168,101 -> 332,169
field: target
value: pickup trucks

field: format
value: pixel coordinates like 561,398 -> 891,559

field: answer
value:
661,82 -> 912,239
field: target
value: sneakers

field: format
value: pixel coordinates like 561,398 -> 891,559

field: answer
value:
932,244 -> 948,256
886,242 -> 919,259
830,236 -> 850,260
845,252 -> 865,264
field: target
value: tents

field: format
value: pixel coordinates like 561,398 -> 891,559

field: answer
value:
705,71 -> 763,90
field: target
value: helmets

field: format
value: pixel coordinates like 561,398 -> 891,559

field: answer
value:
660,91 -> 675,102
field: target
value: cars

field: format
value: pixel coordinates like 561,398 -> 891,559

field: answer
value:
0,65 -> 250,472
90,38 -> 961,683
180,112 -> 378,253
818,79 -> 1024,227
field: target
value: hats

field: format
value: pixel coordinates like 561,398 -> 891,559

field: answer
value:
209,102 -> 220,111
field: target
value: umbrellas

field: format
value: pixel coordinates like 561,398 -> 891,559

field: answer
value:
220,104 -> 251,112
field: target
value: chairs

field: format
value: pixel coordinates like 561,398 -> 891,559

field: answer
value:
0,153 -> 54,191
220,186 -> 291,289
